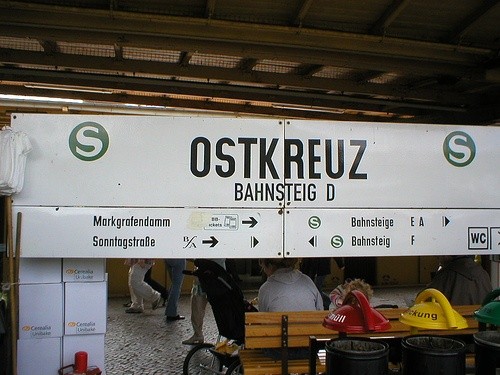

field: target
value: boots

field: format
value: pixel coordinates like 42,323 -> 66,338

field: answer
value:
182,296 -> 207,344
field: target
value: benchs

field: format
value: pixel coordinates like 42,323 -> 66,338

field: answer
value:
241,305 -> 481,375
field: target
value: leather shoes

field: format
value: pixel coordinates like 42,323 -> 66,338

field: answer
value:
165,314 -> 186,322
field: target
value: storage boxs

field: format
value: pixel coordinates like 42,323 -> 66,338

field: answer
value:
62,258 -> 106,282
18,283 -> 64,339
64,282 -> 108,336
18,258 -> 61,284
16,336 -> 62,375
63,334 -> 105,375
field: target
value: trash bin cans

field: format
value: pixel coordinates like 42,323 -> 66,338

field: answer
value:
473,300 -> 500,375
398,288 -> 469,375
320,289 -> 392,375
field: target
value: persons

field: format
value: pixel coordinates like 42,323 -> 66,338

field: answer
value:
299,257 -> 345,310
258,258 -> 324,360
123,258 -> 228,345
416,255 -> 493,344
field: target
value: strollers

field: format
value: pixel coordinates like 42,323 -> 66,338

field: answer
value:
181,258 -> 258,375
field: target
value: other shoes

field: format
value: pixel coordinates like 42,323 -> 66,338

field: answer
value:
123,301 -> 133,307
151,294 -> 162,310
125,308 -> 144,313
